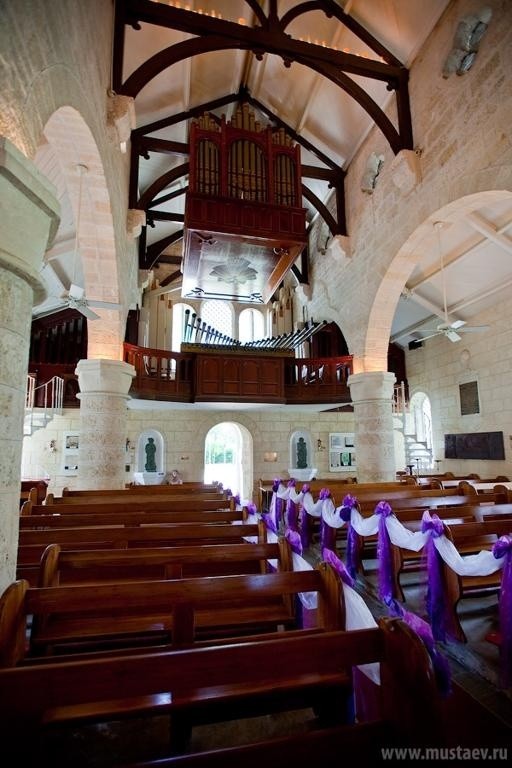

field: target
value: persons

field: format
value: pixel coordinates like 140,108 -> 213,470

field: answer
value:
296,437 -> 307,469
144,437 -> 157,472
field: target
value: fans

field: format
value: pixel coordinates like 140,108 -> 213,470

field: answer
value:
405,218 -> 492,346
35,162 -> 123,326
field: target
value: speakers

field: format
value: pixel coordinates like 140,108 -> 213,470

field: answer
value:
407,338 -> 423,351
